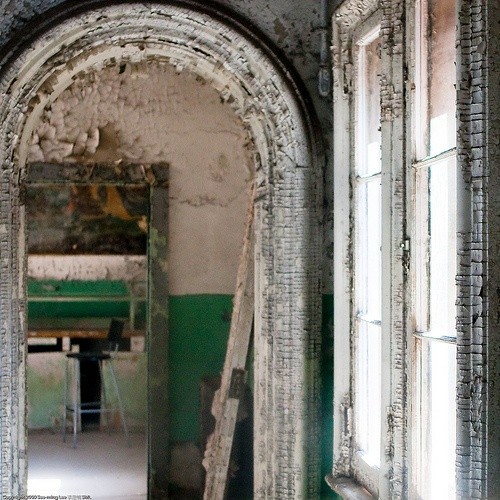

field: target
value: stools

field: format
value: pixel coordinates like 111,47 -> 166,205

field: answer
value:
63,338 -> 131,449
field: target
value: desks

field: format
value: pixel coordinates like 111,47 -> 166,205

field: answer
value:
29,328 -> 148,341
